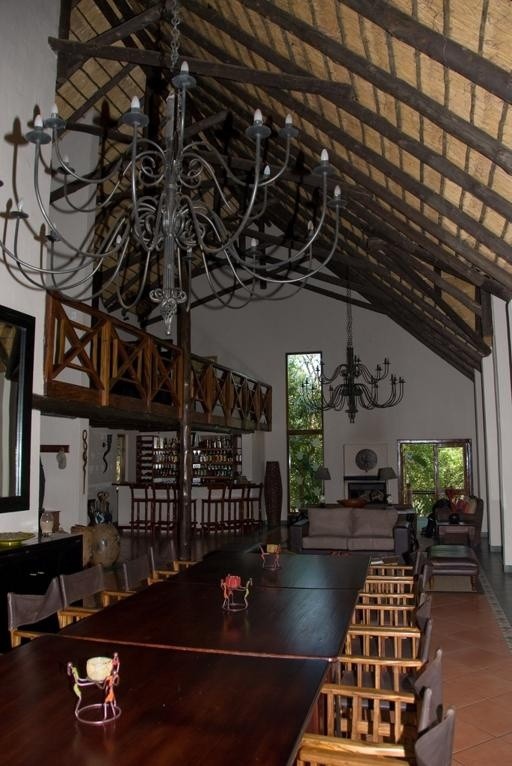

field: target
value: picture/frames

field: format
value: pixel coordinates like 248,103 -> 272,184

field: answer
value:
343,442 -> 390,477
0,305 -> 36,515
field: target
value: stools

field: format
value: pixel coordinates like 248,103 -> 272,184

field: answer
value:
426,545 -> 480,593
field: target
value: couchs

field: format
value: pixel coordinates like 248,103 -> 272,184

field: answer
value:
432,495 -> 485,544
289,507 -> 413,555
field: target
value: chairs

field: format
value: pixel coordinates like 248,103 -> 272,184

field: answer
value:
128,483 -> 153,536
244,483 -> 264,535
150,482 -> 174,539
298,705 -> 459,766
225,483 -> 246,536
123,554 -> 164,594
319,593 -> 436,738
7,576 -> 93,649
59,562 -> 135,612
200,482 -> 226,536
150,540 -> 197,578
301,647 -> 445,758
352,547 -> 436,625
360,538 -> 429,603
173,483 -> 198,535
342,573 -> 428,673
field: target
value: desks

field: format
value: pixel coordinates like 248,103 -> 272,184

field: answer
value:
55,581 -> 358,685
435,520 -> 476,541
167,549 -> 371,590
0,633 -> 332,766
0,531 -> 85,654
299,506 -> 420,553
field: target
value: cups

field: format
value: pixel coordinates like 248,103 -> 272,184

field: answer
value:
86,656 -> 113,682
265,543 -> 277,555
226,576 -> 241,588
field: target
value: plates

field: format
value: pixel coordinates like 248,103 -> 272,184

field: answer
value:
0,531 -> 37,545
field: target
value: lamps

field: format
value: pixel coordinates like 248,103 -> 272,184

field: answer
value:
0,0 -> 349,335
301,285 -> 405,425
314,467 -> 332,503
377,466 -> 399,505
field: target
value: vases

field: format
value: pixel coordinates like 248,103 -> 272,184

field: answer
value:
88,522 -> 121,568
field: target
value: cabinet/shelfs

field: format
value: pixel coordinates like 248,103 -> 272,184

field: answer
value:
135,434 -> 242,479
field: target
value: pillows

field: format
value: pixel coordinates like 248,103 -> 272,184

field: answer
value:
456,495 -> 471,511
465,498 -> 478,514
